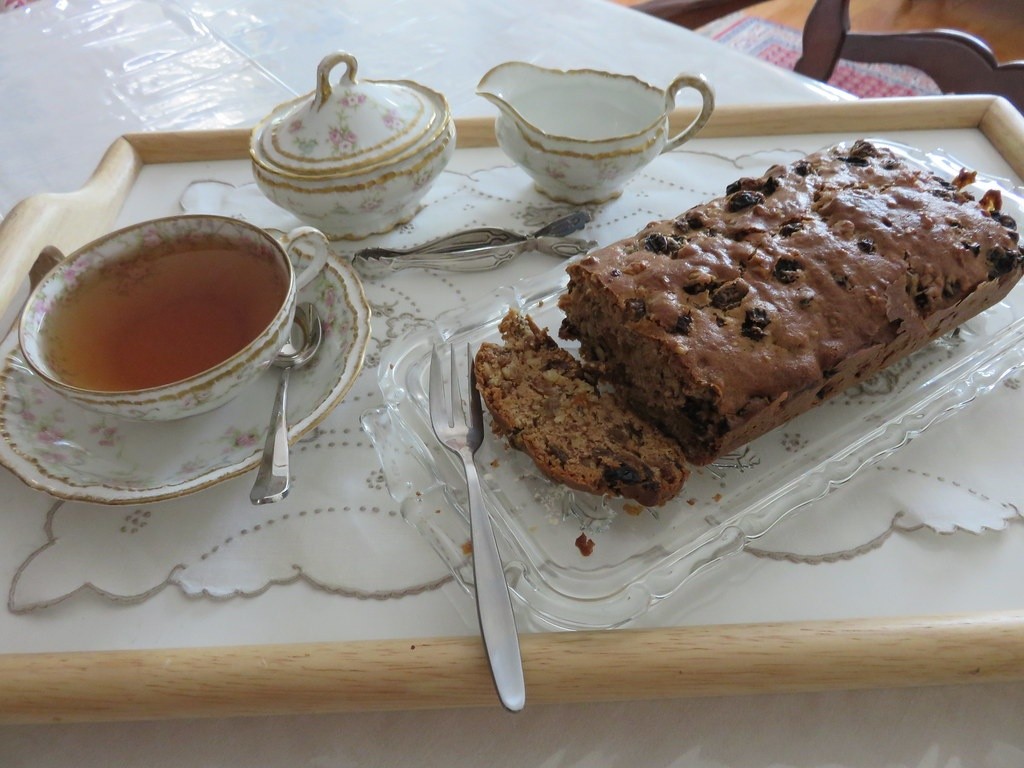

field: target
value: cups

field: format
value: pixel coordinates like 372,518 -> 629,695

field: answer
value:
476,61 -> 716,206
16,213 -> 329,421
248,52 -> 457,240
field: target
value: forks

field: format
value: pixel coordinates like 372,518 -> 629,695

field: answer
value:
429,342 -> 538,713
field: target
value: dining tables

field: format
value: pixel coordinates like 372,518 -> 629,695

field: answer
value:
1,0 -> 1024,768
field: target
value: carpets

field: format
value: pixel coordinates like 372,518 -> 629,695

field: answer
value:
712,15 -> 956,99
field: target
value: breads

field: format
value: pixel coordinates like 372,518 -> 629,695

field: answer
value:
466,138 -> 1024,504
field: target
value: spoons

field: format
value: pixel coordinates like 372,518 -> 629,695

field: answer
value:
250,302 -> 322,504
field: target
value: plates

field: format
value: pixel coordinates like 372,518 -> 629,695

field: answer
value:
0,228 -> 372,507
363,138 -> 1024,632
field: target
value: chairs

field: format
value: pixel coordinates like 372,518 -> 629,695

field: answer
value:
634,1 -> 1024,117
